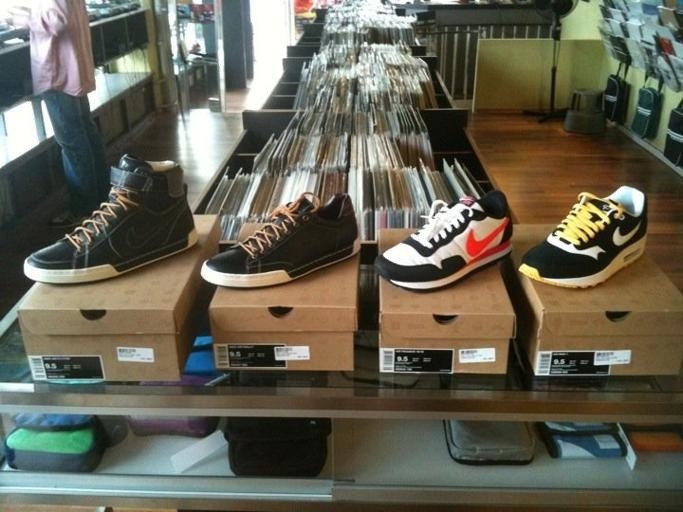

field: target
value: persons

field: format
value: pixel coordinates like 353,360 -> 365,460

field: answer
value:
7,0 -> 111,228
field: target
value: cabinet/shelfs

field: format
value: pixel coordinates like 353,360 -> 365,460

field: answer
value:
1,261 -> 682,507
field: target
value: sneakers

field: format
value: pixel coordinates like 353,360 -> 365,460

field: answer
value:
24,154 -> 198,285
374,189 -> 513,292
48,208 -> 88,229
518,186 -> 647,290
200,190 -> 362,289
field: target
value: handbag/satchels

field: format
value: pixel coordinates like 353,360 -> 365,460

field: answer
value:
184,337 -> 222,381
5,414 -> 106,472
602,62 -> 628,124
125,374 -> 217,438
663,98 -> 682,167
630,71 -> 662,140
222,414 -> 331,478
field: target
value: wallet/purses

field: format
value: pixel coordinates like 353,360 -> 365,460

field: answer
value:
443,418 -> 535,464
538,421 -> 628,460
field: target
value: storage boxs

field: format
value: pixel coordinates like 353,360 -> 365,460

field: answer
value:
376,225 -> 516,376
208,221 -> 362,372
500,223 -> 682,379
18,213 -> 224,383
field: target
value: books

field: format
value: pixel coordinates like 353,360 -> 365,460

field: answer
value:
292,0 -> 439,110
205,109 -> 488,240
600,1 -> 683,92
